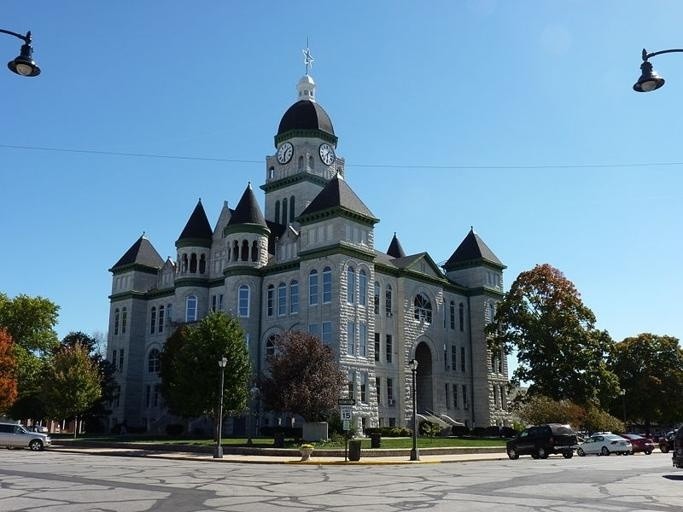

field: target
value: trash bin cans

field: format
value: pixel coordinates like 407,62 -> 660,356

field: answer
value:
371,432 -> 381,448
348,440 -> 361,461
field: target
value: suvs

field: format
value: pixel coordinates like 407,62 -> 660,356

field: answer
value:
506,421 -> 578,460
672,425 -> 682,470
0,423 -> 52,452
657,428 -> 677,453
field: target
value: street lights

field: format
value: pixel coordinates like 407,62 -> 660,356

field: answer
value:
0,26 -> 40,78
632,48 -> 682,93
213,355 -> 228,458
407,360 -> 421,462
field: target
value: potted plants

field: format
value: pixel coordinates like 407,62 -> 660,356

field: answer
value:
299,443 -> 314,461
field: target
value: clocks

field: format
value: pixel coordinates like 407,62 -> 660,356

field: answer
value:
276,141 -> 294,165
319,142 -> 335,166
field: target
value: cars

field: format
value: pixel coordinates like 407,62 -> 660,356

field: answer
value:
620,434 -> 655,457
28,426 -> 47,432
576,432 -> 633,457
629,431 -> 669,443
